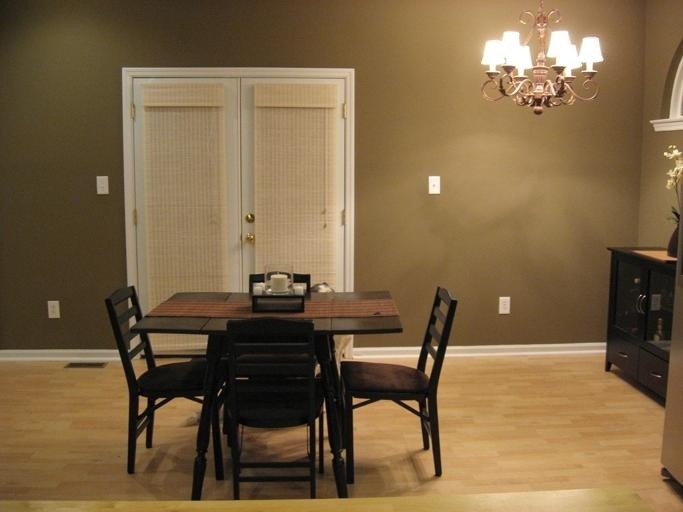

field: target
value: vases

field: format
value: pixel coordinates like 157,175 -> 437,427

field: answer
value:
666,210 -> 680,259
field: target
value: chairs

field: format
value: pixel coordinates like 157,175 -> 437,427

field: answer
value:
103,284 -> 229,481
249,270 -> 311,293
340,284 -> 458,485
224,315 -> 324,499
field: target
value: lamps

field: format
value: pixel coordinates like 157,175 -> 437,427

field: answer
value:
480,1 -> 605,116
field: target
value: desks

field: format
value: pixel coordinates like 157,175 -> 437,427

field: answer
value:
131,291 -> 403,500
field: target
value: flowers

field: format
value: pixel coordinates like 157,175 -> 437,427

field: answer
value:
664,145 -> 683,217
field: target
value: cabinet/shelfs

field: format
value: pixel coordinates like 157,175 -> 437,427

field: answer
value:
605,246 -> 677,409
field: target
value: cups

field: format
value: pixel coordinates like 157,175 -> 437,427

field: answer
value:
270,274 -> 287,290
